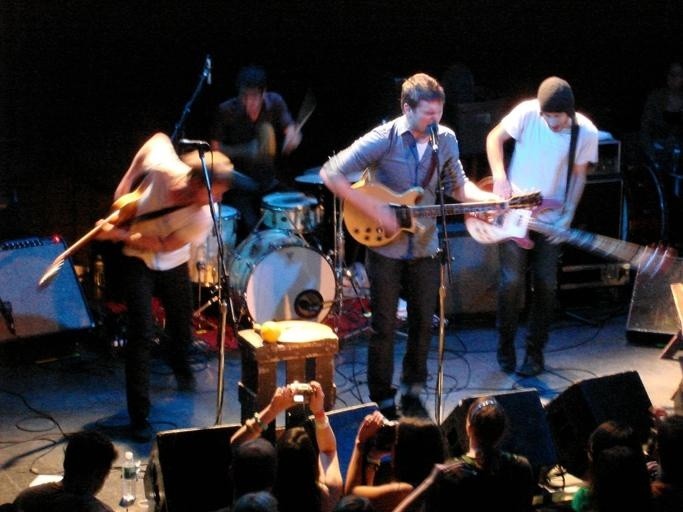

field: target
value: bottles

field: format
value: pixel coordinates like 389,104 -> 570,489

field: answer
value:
119,452 -> 137,505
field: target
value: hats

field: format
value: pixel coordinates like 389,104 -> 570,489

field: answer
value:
537,75 -> 574,111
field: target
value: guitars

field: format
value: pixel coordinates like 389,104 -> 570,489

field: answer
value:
345,183 -> 543,247
464,175 -> 675,280
38,174 -> 152,290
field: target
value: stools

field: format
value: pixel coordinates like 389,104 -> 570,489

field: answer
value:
234,318 -> 343,450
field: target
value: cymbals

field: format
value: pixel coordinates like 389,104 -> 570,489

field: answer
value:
297,164 -> 364,185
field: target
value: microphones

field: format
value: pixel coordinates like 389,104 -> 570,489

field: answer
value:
179,135 -> 210,150
205,56 -> 213,85
2,300 -> 16,334
428,123 -> 440,153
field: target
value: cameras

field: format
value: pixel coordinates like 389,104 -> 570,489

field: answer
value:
288,382 -> 313,406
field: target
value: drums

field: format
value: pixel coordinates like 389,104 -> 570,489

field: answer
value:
188,204 -> 239,286
224,229 -> 337,323
260,192 -> 326,233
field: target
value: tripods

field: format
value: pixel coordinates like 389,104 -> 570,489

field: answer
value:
326,230 -> 369,318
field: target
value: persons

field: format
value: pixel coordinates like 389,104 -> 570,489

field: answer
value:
211,68 -> 301,242
91,131 -> 234,444
484,76 -> 600,376
11,429 -> 119,512
319,72 -> 500,420
208,379 -> 683,512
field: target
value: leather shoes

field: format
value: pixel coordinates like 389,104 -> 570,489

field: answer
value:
496,341 -> 543,376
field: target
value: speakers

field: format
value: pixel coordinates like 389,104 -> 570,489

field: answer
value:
442,387 -> 547,460
298,402 -> 382,482
145,426 -> 286,512
626,254 -> 683,344
545,370 -> 653,459
